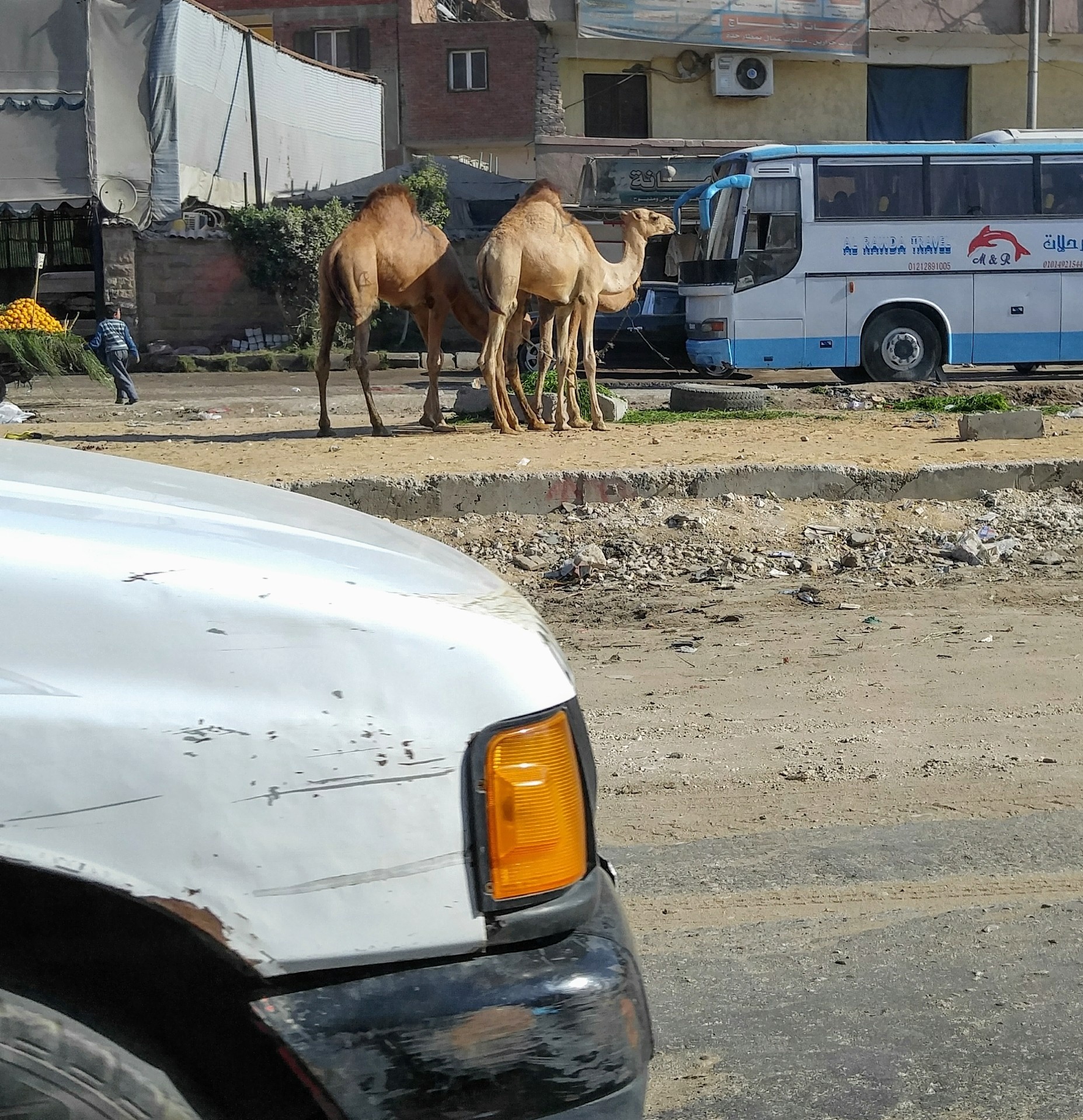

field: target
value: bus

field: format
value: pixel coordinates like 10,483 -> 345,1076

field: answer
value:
672,127 -> 1083,382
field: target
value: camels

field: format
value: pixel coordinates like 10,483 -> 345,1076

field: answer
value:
316,184 -> 539,438
477,178 -> 677,430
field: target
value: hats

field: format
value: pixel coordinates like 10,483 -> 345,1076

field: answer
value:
1045,195 -> 1054,208
878,196 -> 888,211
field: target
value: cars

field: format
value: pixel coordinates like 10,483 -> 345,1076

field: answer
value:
0,429 -> 663,1119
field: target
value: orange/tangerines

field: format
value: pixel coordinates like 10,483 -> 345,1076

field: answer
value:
0,298 -> 61,331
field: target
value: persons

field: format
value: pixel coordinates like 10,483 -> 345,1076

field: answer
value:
739,218 -> 798,284
83,305 -> 140,405
819,182 -> 1081,217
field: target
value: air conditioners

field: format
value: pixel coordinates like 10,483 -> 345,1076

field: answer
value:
183,212 -> 207,231
711,52 -> 775,98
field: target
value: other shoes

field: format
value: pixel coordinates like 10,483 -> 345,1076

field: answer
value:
115,399 -> 124,404
125,399 -> 139,405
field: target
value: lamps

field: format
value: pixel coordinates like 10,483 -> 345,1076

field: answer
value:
661,155 -> 679,177
